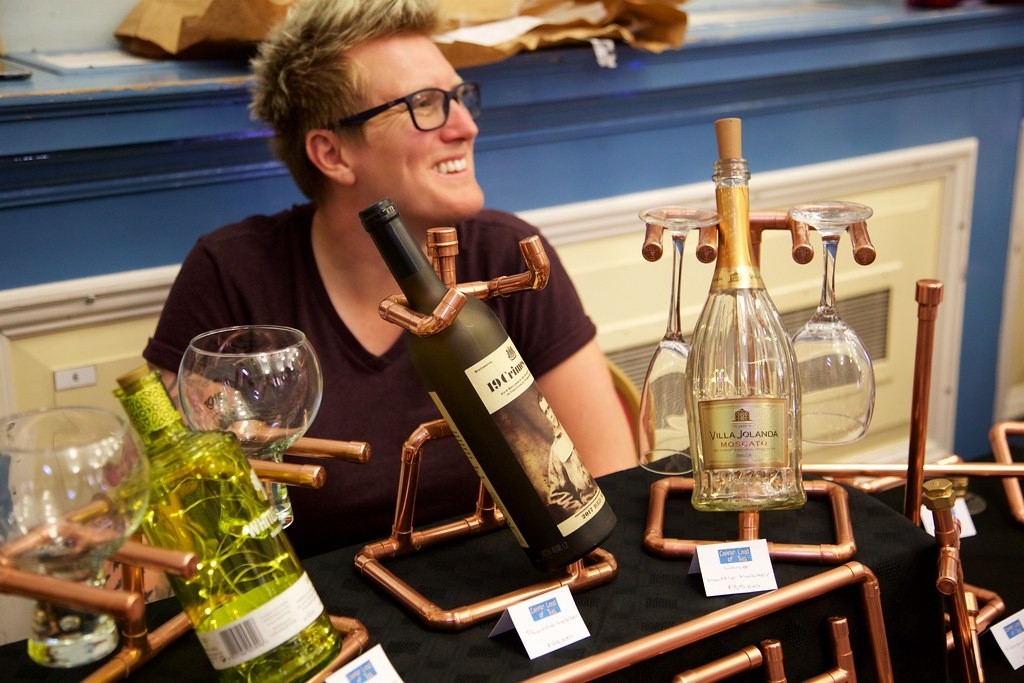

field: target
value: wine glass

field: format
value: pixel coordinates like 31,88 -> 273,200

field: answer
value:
178,324 -> 323,538
788,201 -> 876,445
636,205 -> 724,475
0,405 -> 152,668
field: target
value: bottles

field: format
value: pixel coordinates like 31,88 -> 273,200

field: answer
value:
358,196 -> 618,571
113,366 -> 341,683
684,118 -> 807,512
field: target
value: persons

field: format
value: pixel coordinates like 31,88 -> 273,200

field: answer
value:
104,0 -> 638,558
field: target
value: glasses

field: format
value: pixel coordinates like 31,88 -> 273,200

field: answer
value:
331,81 -> 483,133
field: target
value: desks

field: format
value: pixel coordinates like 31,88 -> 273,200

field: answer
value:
0,434 -> 1024,683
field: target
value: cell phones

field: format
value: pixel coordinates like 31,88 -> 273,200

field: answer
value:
0,60 -> 32,80
